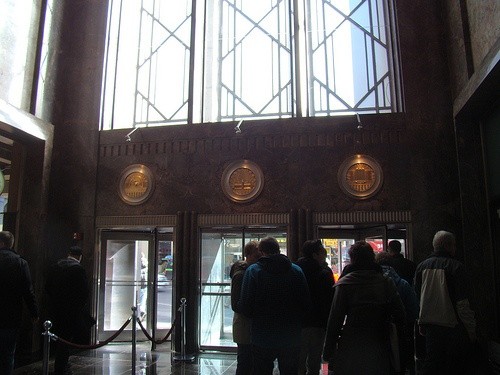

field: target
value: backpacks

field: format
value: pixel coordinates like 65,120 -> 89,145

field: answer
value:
265,255 -> 310,327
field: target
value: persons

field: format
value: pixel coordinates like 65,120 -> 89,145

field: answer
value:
413,230 -> 480,375
0,231 -> 40,375
46,247 -> 90,375
230,237 -> 418,375
